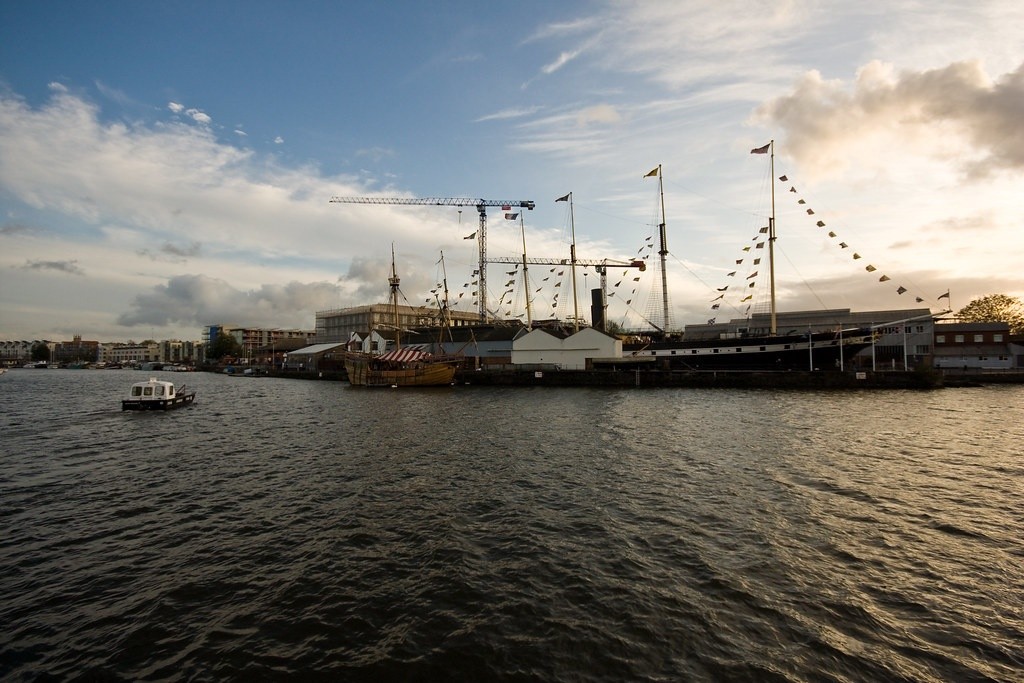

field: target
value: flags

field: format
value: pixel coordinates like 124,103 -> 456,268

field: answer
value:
751,144 -> 770,154
416,168 -> 951,325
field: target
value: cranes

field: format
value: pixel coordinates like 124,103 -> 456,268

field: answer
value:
486,256 -> 647,332
328,197 -> 536,325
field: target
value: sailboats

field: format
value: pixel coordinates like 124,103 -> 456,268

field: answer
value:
342,240 -> 461,389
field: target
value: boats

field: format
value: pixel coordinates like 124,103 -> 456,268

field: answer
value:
121,377 -> 197,411
556,139 -> 955,372
